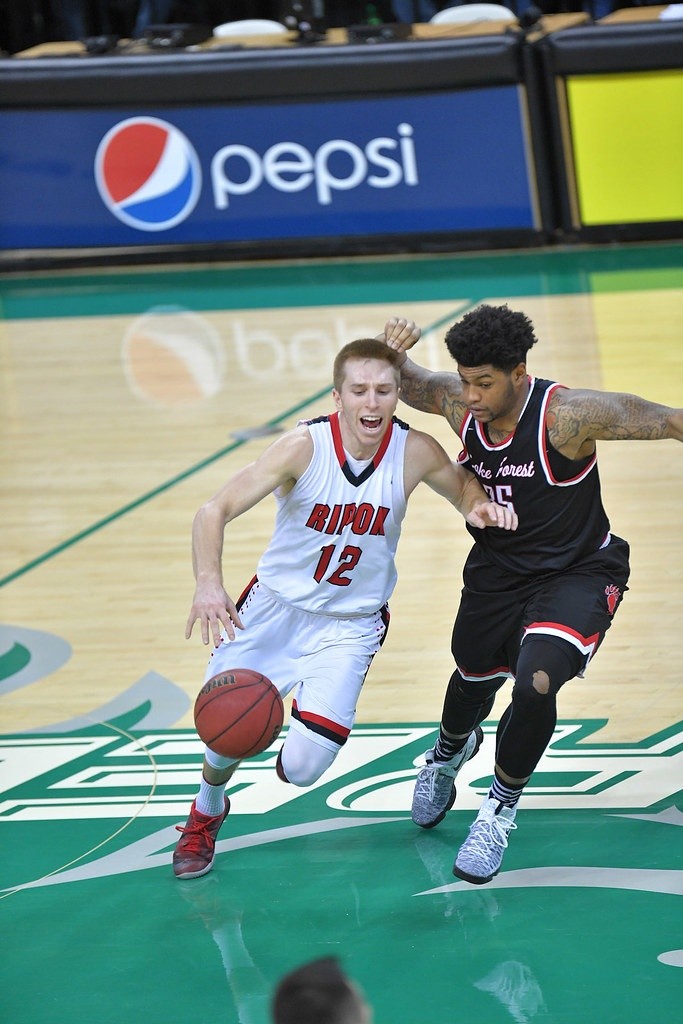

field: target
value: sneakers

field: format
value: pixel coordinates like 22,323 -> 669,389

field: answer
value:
411,725 -> 483,828
172,795 -> 231,880
453,794 -> 516,885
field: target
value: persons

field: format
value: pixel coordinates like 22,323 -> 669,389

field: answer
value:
171,337 -> 519,882
380,304 -> 683,885
270,956 -> 370,1024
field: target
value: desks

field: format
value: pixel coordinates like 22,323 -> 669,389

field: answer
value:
9,0 -> 683,58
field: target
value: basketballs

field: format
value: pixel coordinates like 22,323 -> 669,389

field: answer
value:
191,668 -> 289,759
